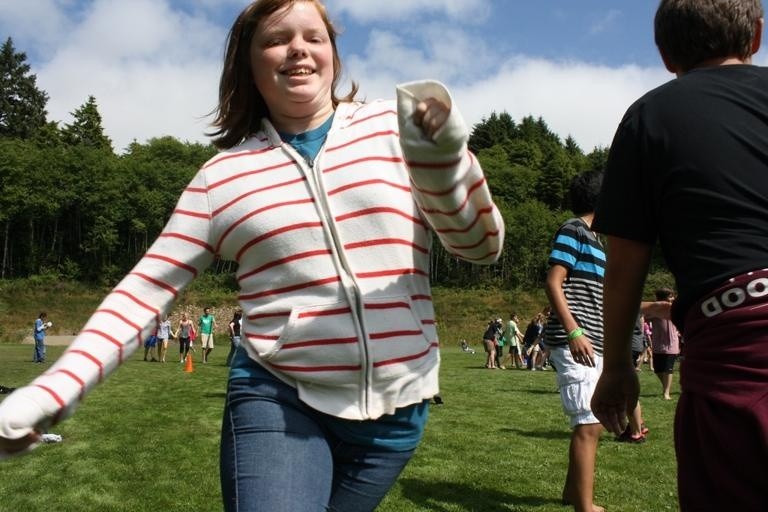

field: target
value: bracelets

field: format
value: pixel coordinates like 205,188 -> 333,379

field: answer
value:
567,327 -> 584,338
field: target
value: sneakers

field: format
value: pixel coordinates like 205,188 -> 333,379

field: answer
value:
617,418 -> 649,441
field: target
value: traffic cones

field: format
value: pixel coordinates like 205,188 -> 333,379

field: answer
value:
184,354 -> 192,372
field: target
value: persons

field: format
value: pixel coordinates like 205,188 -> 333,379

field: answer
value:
482,305 -> 556,370
589,0 -> 768,511
33,313 -> 48,362
618,285 -> 682,443
0,0 -> 505,512
143,308 -> 242,364
540,169 -> 608,511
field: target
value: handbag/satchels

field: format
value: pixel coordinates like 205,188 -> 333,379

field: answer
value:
190,330 -> 195,341
522,343 -> 534,357
